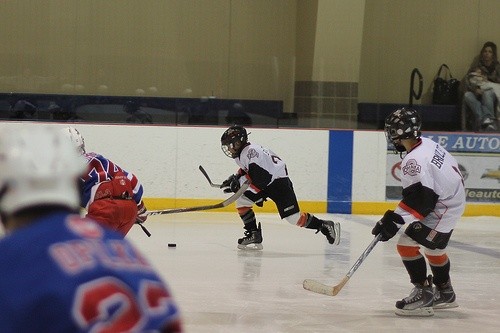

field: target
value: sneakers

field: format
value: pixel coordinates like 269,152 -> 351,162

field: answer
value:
320,220 -> 340,245
482,117 -> 496,130
393,287 -> 435,316
237,222 -> 263,250
433,280 -> 459,308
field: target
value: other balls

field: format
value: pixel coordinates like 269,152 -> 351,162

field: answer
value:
168,244 -> 176,247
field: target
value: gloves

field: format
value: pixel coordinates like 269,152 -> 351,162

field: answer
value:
135,200 -> 147,222
372,210 -> 404,242
220,174 -> 240,192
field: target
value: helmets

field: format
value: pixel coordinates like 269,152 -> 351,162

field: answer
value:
220,125 -> 248,158
0,126 -> 82,212
384,105 -> 422,152
63,127 -> 84,148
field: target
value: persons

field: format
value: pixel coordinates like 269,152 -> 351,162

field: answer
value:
219,125 -> 336,246
61,125 -> 148,240
462,42 -> 500,133
371,107 -> 466,310
1,123 -> 185,333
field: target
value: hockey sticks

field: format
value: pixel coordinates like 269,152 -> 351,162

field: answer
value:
199,165 -> 232,188
303,232 -> 383,296
147,183 -> 249,216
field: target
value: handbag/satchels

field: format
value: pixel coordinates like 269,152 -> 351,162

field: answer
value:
432,64 -> 460,104
